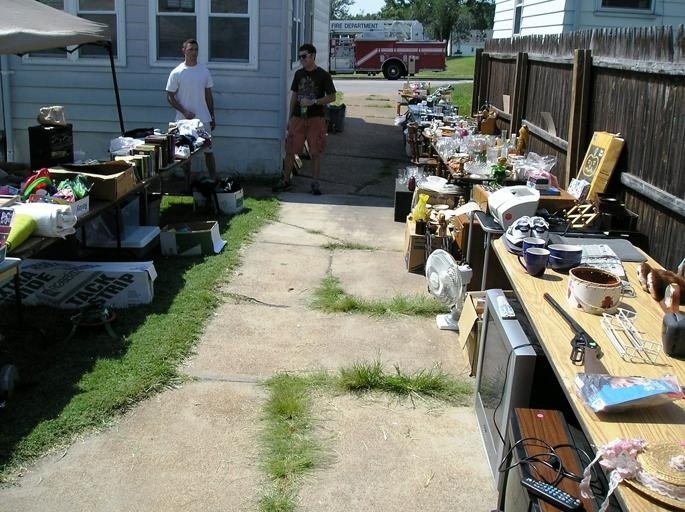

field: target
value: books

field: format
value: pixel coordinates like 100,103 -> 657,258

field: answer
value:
110,134 -> 176,181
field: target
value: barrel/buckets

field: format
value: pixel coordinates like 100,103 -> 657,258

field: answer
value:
216,188 -> 243,215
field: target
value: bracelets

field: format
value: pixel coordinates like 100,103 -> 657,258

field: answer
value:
212,116 -> 214,120
312,99 -> 317,105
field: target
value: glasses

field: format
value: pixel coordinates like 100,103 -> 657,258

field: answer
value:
298,53 -> 309,59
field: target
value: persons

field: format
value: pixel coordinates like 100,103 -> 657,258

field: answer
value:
272,43 -> 336,195
165,39 -> 216,180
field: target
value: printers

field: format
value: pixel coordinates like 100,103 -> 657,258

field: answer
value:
487,185 -> 540,232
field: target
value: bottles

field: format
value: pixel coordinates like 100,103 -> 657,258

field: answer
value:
397,166 -> 435,191
497,124 -> 529,156
301,97 -> 308,120
413,194 -> 429,224
438,213 -> 447,236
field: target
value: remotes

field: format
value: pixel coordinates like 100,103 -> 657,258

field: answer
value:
520,474 -> 583,512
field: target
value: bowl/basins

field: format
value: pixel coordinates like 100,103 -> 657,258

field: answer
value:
548,243 -> 583,272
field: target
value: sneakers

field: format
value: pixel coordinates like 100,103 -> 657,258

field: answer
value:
311,182 -> 322,195
273,180 -> 293,192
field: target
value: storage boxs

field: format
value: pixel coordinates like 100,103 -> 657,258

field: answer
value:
159,219 -> 227,256
404,215 -> 443,271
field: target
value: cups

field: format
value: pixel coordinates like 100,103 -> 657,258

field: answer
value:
517,247 -> 551,278
523,236 -> 546,263
415,220 -> 427,234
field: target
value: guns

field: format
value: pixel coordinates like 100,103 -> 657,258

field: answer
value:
543,291 -> 609,374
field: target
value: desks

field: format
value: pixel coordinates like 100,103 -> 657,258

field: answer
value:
0,145 -> 214,362
408,95 -> 685,511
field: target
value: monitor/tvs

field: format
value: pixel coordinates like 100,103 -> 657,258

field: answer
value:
496,407 -> 623,512
474,289 -> 572,491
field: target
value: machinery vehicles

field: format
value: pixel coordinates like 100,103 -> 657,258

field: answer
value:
329,20 -> 447,80
